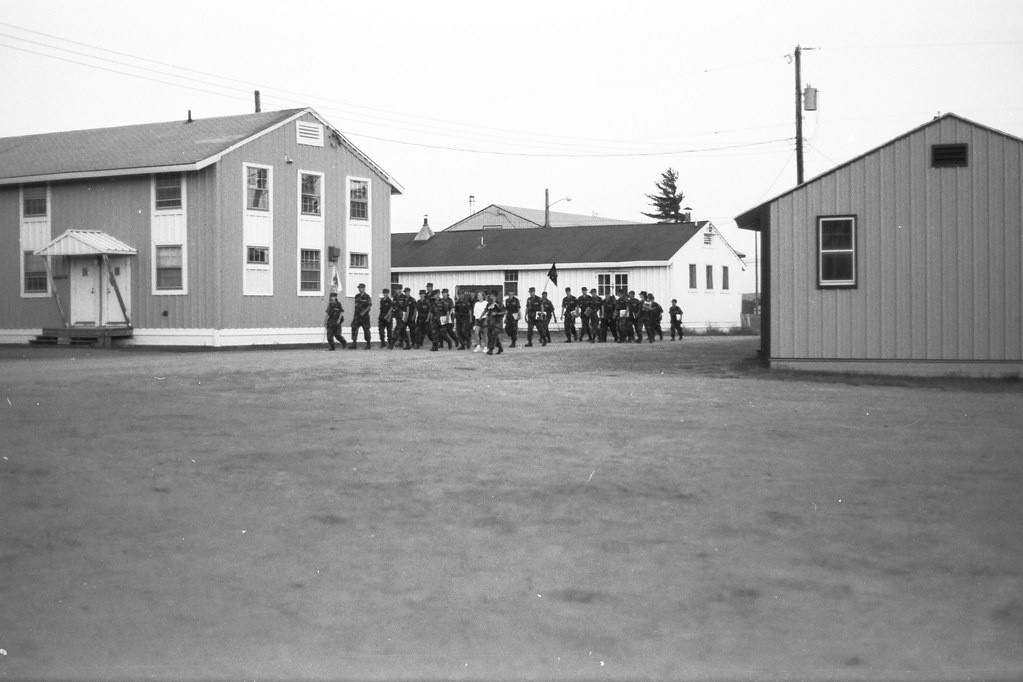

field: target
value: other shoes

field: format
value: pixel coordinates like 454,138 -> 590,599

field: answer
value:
348,346 -> 356,349
483,347 -> 489,352
364,346 -> 371,349
473,345 -> 482,352
328,348 -> 335,350
380,339 -> 471,351
487,338 -> 551,355
343,342 -> 346,349
564,334 -> 682,343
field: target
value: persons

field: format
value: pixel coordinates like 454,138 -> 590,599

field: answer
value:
322,282 -> 664,354
668,299 -> 684,342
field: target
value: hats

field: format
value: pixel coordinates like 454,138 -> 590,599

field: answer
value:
396,283 -> 515,297
542,292 -> 547,295
582,287 -> 654,301
566,288 -> 570,292
357,284 -> 365,288
528,288 -> 534,292
330,293 -> 337,297
382,289 -> 389,293
671,299 -> 677,303
